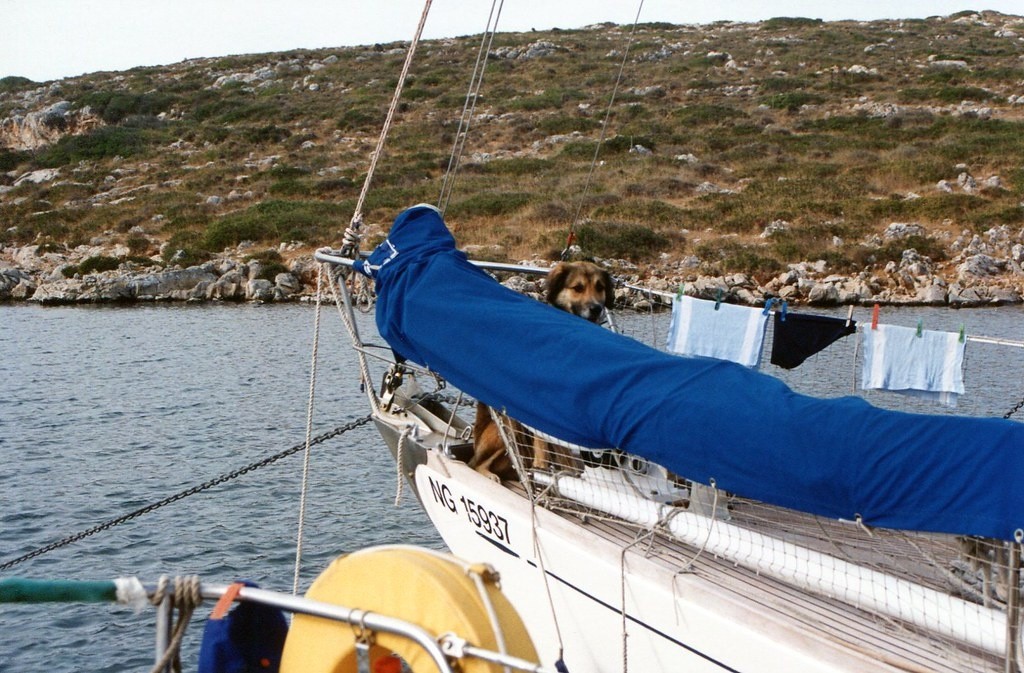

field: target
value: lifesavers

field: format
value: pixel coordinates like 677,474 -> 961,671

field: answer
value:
279,539 -> 543,672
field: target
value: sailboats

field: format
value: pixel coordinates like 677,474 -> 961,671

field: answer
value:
321,0 -> 1024,673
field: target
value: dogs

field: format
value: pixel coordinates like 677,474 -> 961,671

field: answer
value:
467,261 -> 614,486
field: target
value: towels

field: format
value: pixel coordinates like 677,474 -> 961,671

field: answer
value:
665,294 -> 771,372
861,322 -> 970,409
